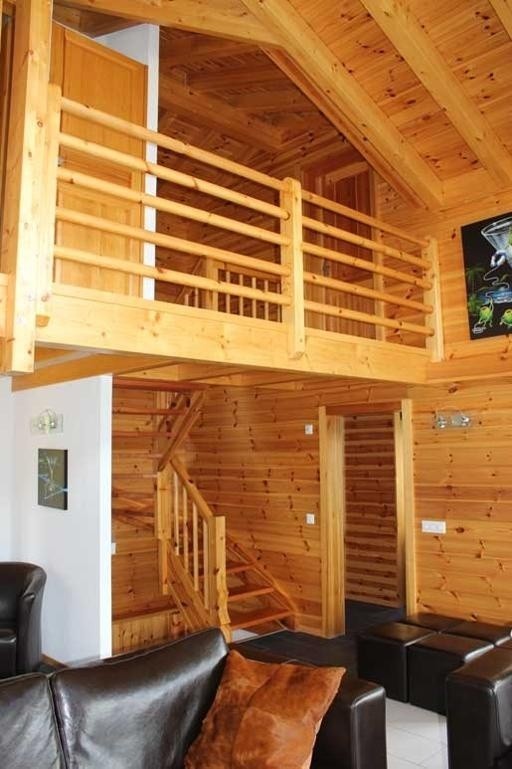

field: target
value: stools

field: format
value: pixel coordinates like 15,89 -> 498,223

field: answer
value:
355,611 -> 512,716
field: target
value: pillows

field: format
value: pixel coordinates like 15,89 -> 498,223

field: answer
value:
186,646 -> 348,769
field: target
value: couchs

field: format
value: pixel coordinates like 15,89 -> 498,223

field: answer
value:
0,627 -> 394,769
437,633 -> 512,768
0,560 -> 46,676
